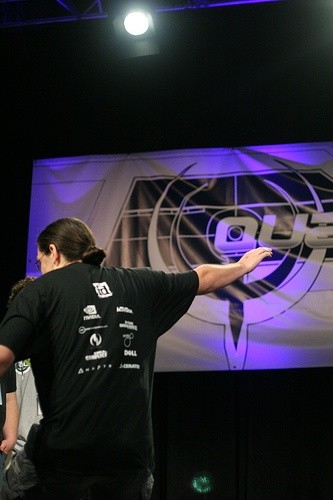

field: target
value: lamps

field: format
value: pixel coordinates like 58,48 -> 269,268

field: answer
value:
110,6 -> 162,64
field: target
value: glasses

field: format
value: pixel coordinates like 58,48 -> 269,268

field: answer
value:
34,248 -> 64,271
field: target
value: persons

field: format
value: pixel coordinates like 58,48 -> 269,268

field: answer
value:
1,215 -> 274,499
